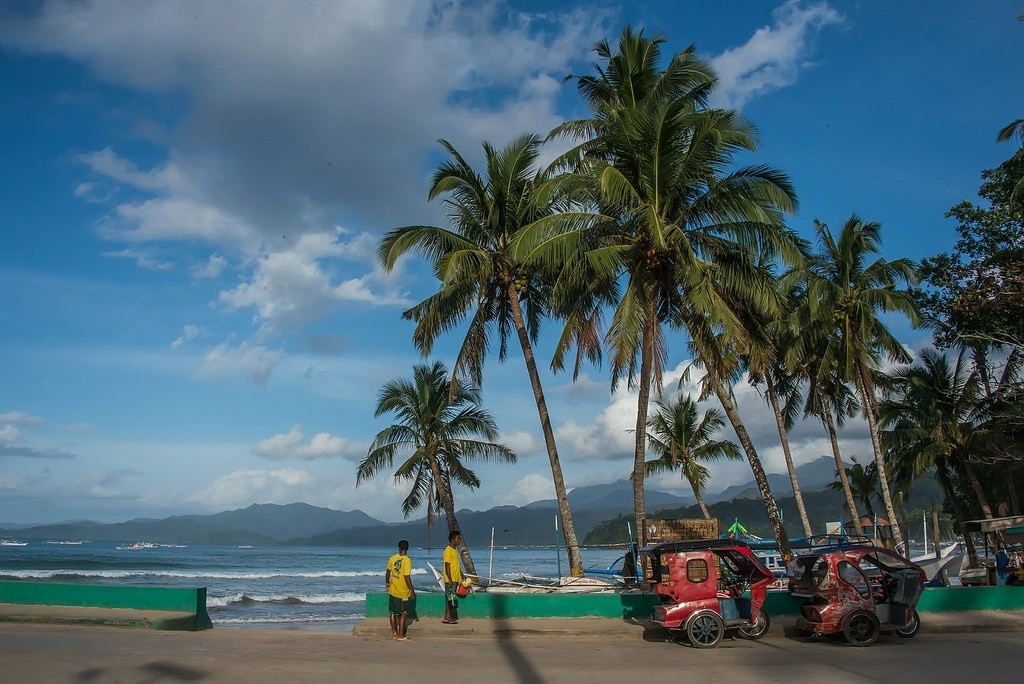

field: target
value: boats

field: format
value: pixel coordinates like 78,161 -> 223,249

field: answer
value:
115,540 -> 187,550
427,534 -> 1011,593
0,539 -> 28,546
43,541 -> 83,545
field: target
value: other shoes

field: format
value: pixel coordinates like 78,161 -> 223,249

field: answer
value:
442,619 -> 458,624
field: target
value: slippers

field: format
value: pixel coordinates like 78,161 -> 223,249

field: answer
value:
396,636 -> 412,641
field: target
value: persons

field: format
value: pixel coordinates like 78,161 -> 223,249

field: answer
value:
385,540 -> 415,641
443,531 -> 467,621
1009,550 -> 1024,569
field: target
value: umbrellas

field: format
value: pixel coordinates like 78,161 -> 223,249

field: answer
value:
843,514 -> 891,534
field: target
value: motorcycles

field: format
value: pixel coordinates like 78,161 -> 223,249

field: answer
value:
639,538 -> 780,648
782,534 -> 928,647
